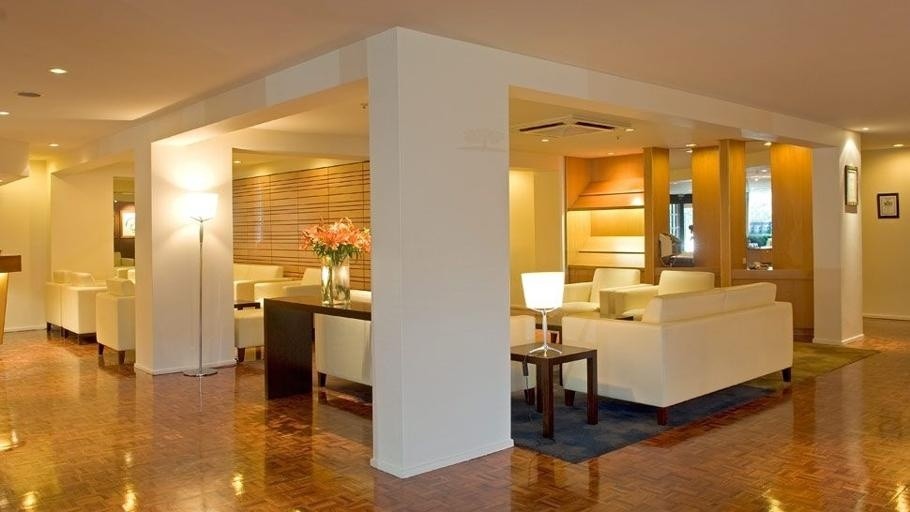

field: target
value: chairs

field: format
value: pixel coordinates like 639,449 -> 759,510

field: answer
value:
39,251 -> 321,373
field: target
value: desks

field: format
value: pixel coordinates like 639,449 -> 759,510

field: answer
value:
259,288 -> 373,403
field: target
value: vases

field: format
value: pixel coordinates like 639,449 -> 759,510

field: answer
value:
318,253 -> 351,311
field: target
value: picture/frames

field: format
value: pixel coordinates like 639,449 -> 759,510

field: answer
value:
842,162 -> 859,207
876,192 -> 901,219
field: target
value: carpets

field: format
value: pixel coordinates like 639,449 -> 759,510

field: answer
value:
507,338 -> 881,469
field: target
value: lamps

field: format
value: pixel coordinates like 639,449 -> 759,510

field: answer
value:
182,190 -> 220,379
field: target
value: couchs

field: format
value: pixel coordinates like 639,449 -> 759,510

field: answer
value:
535,268 -> 642,346
611,270 -> 718,321
562,261 -> 793,427
310,306 -> 539,408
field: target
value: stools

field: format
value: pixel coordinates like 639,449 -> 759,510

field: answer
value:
509,336 -> 598,440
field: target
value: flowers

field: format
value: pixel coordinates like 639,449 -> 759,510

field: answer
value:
295,214 -> 372,307
